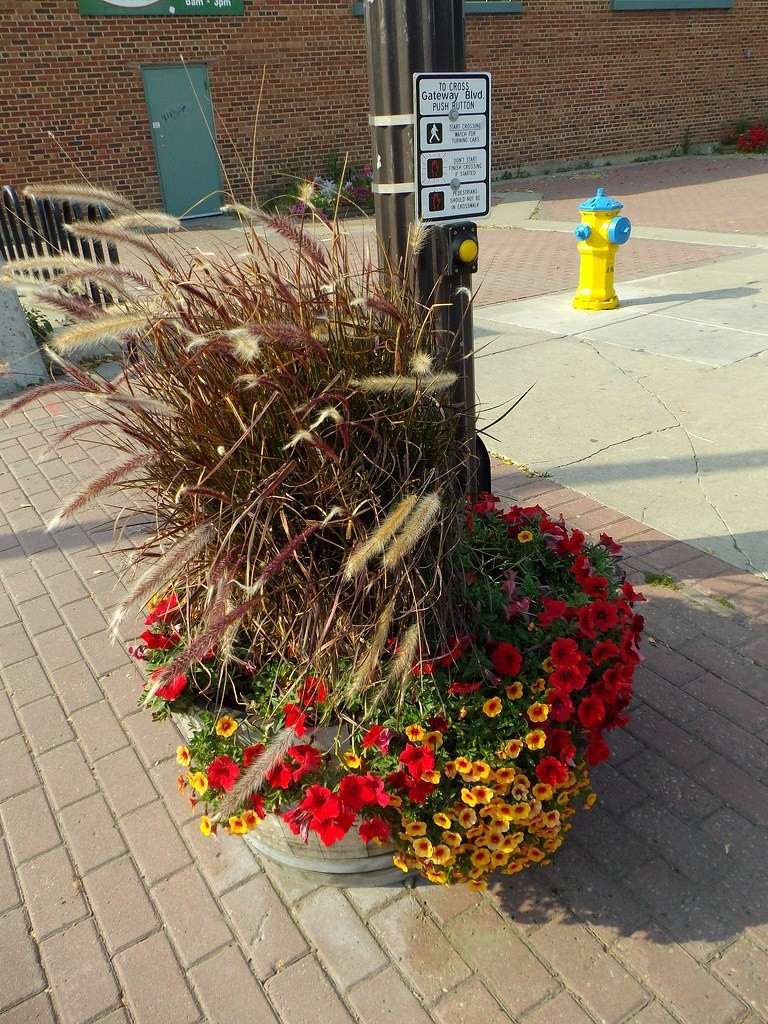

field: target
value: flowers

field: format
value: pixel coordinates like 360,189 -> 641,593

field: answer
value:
261,141 -> 374,219
126,491 -> 647,890
717,111 -> 768,154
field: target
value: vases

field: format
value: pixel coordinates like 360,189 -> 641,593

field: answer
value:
198,704 -> 426,887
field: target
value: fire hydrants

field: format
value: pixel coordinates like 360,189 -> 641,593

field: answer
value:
573,185 -> 632,311
